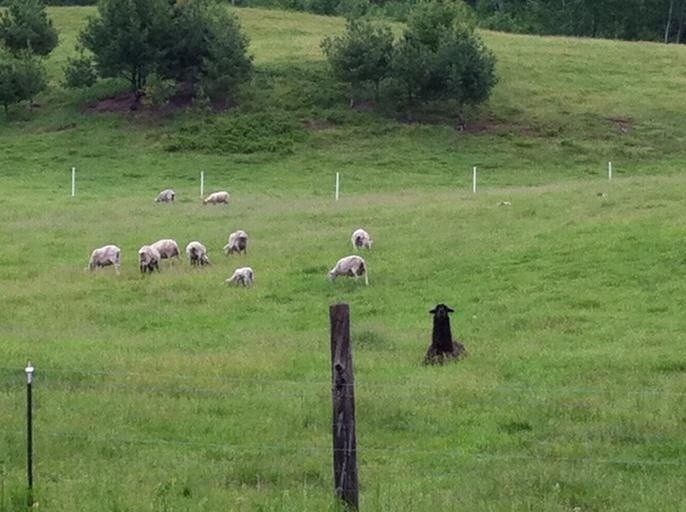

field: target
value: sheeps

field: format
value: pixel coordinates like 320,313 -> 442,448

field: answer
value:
154,189 -> 175,204
351,229 -> 373,252
203,191 -> 230,205
329,256 -> 368,287
82,245 -> 121,276
150,239 -> 182,269
223,230 -> 247,257
186,241 -> 210,268
138,245 -> 160,272
221,267 -> 254,289
